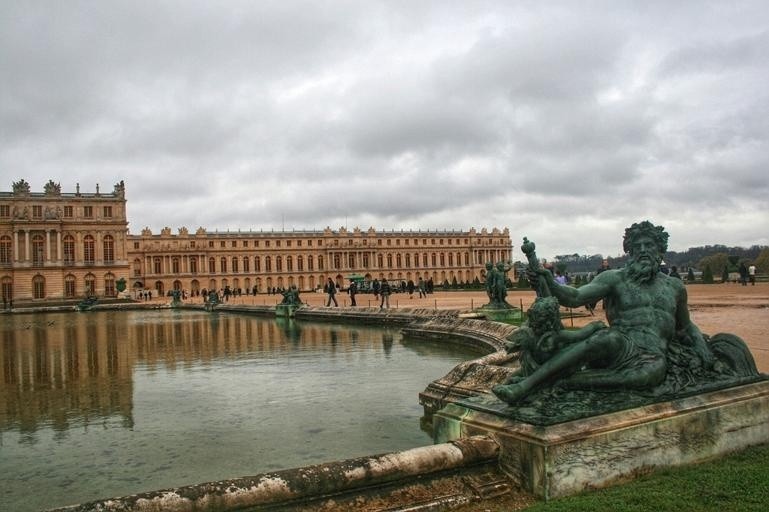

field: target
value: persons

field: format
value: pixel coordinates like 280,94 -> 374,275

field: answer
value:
486,261 -> 511,304
739,262 -> 747,286
748,263 -> 756,285
491,221 -> 709,400
504,296 -> 606,385
137,276 -> 434,307
554,271 -> 568,285
